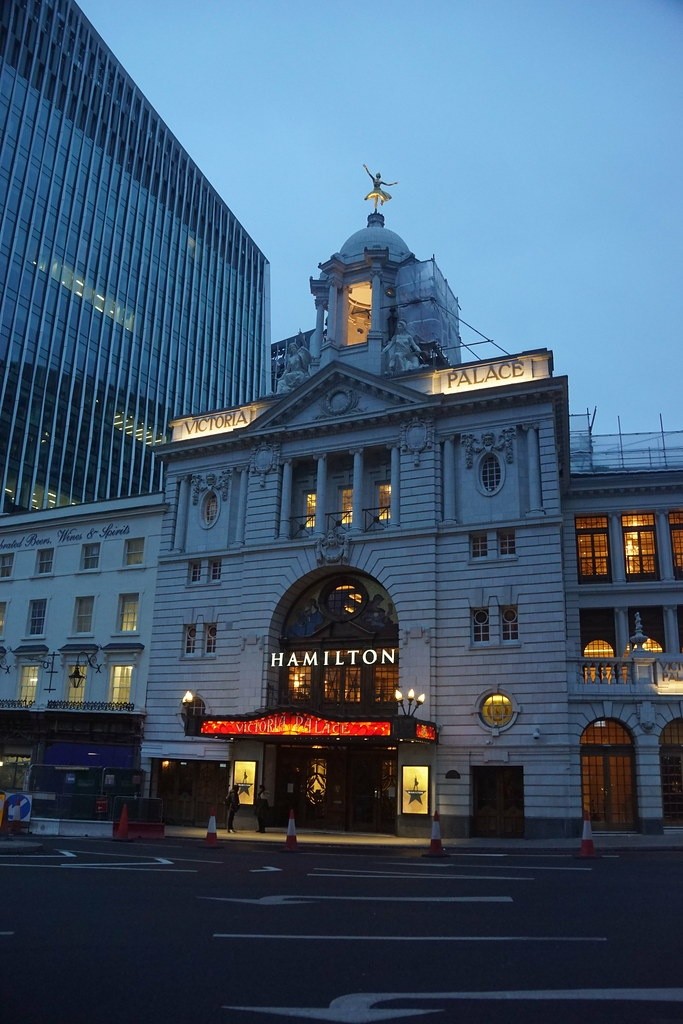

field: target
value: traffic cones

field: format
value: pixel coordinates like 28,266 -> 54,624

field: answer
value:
420,811 -> 450,857
197,807 -> 218,848
110,803 -> 132,842
573,811 -> 601,858
278,808 -> 305,852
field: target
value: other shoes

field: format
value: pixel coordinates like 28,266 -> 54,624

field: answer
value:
228,828 -> 236,832
256,830 -> 261,832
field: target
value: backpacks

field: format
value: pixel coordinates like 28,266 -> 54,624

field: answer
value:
223,793 -> 231,807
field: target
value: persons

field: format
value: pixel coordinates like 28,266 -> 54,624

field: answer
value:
254,785 -> 270,833
229,785 -> 239,833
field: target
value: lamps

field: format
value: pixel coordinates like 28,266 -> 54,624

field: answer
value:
182,690 -> 193,715
0,646 -> 12,674
394,689 -> 426,719
68,651 -> 102,688
625,540 -> 639,560
